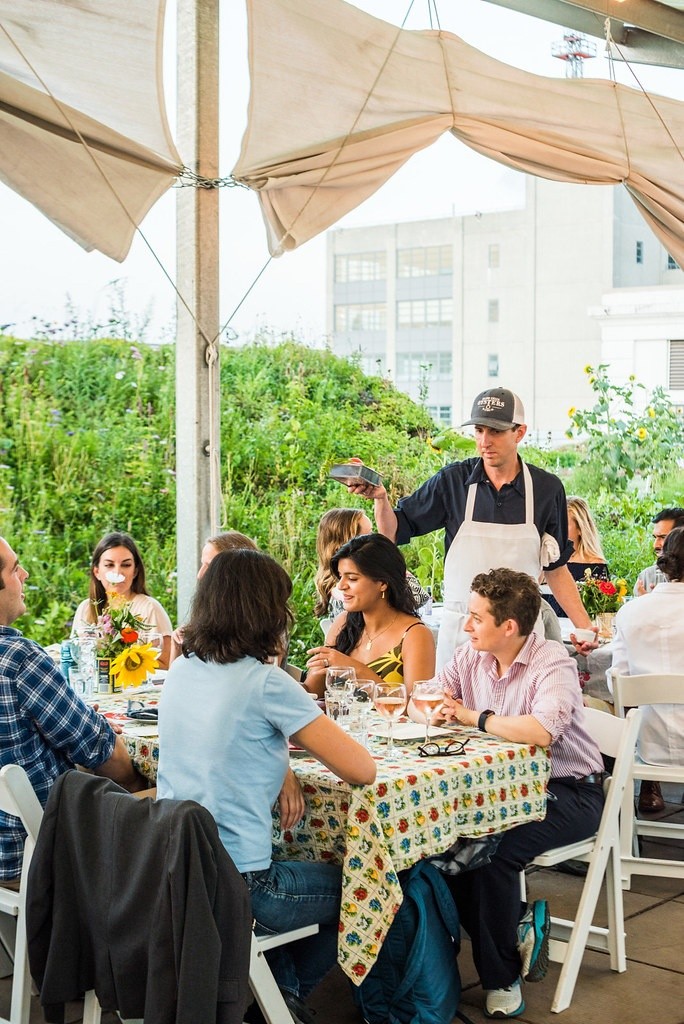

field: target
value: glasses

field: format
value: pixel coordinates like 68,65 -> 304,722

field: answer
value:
416,739 -> 471,757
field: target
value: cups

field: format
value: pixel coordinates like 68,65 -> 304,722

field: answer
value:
610,617 -> 617,635
573,628 -> 596,643
622,596 -> 636,604
439,580 -> 445,598
324,690 -> 354,727
68,666 -> 92,700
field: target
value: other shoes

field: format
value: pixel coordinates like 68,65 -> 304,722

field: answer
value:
241,993 -> 317,1024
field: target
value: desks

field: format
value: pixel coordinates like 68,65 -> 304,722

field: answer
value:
419,603 -> 618,705
43,643 -> 551,987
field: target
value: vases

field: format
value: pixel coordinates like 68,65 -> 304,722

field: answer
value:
598,613 -> 616,638
95,656 -> 123,694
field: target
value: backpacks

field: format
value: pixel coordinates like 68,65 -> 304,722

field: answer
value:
348,854 -> 471,1024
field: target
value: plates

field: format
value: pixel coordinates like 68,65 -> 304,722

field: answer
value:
134,718 -> 158,725
369,723 -> 456,741
289,749 -> 306,753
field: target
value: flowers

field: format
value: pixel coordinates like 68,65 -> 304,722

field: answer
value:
82,572 -> 160,687
577,567 -> 629,620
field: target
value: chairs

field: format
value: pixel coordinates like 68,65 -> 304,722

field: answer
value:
27,770 -> 320,1023
610,668 -> 684,891
1,765 -> 145,1024
520,707 -> 642,1014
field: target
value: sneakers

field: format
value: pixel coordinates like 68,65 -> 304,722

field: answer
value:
482,975 -> 526,1019
517,898 -> 551,985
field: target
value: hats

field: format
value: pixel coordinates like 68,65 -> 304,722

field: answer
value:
461,388 -> 525,431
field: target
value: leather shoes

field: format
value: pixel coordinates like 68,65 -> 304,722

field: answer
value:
638,780 -> 665,812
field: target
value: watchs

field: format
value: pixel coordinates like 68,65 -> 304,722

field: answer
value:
478,709 -> 495,732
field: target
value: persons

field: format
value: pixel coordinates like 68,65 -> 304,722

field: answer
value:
0,538 -> 157,893
317,507 -> 430,625
155,550 -> 376,1024
65,532 -> 172,670
631,508 -> 684,810
407,567 -> 605,1018
568,495 -> 606,563
348,387 -> 599,677
168,531 -> 288,672
298,534 -> 436,703
605,527 -> 684,768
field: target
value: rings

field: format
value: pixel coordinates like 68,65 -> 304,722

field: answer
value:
363,492 -> 370,496
323,659 -> 328,666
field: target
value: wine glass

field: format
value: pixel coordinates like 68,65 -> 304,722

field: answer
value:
70,626 -> 98,702
374,683 -> 407,766
137,632 -> 163,693
325,665 -> 356,731
412,681 -> 445,755
343,679 -> 376,751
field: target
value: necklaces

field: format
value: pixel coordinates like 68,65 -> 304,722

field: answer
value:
363,609 -> 401,651
